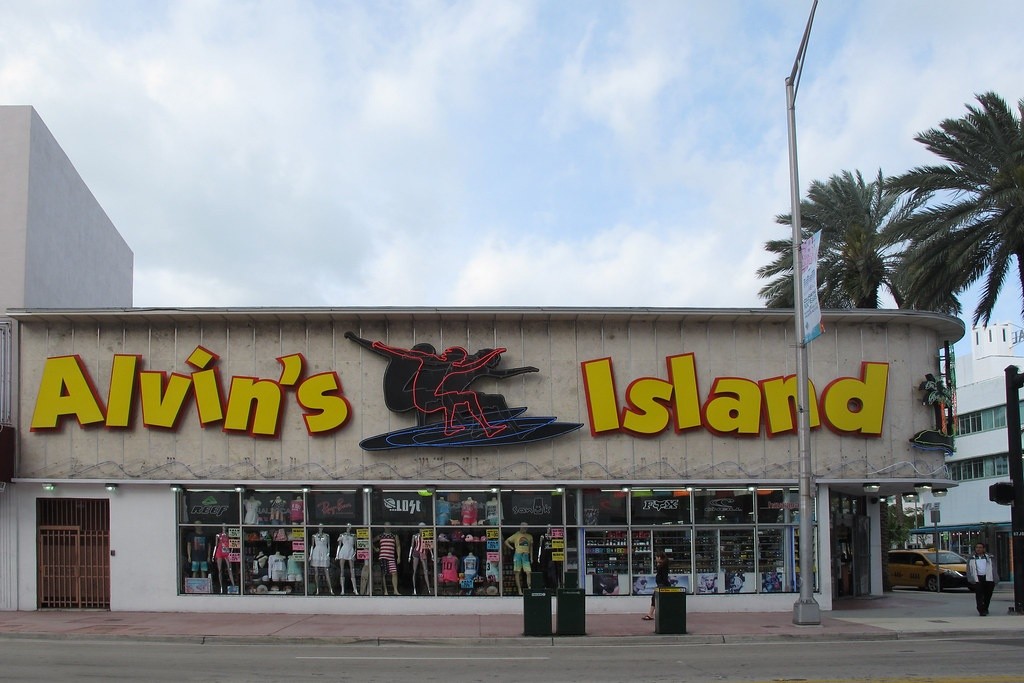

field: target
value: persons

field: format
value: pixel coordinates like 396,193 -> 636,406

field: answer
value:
253,551 -> 269,582
505,522 -> 532,593
334,523 -> 359,595
463,553 -> 480,578
286,552 -> 302,581
187,521 -> 210,578
289,496 -> 305,525
440,553 -> 458,582
966,544 -> 1000,616
309,523 -> 334,595
243,496 -> 262,525
485,497 -> 505,526
641,551 -> 673,620
371,521 -> 402,595
435,497 -> 452,526
268,552 -> 287,581
460,497 -> 478,526
407,522 -> 434,594
538,524 -> 559,589
269,496 -> 287,525
212,522 -> 235,593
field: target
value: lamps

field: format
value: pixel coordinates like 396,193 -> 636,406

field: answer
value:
300,486 -> 313,492
902,492 -> 919,502
41,483 -> 56,491
105,483 -> 119,491
363,486 -> 374,493
233,485 -> 247,492
425,486 -> 436,493
169,484 -> 184,492
489,485 -> 502,494
931,488 -> 948,497
913,483 -> 933,493
862,483 -> 881,493
621,486 -> 759,493
555,485 -> 568,492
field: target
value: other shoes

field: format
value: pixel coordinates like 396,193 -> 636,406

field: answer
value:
980,608 -> 988,616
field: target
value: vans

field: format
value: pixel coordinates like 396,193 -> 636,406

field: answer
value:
888,548 -> 976,591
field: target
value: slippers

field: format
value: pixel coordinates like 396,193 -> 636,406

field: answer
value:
642,615 -> 654,620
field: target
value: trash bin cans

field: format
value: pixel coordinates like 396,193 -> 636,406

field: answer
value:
655,587 -> 686,634
524,588 -> 585,636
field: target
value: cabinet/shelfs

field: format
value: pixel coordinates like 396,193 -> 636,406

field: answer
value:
585,535 -> 782,574
